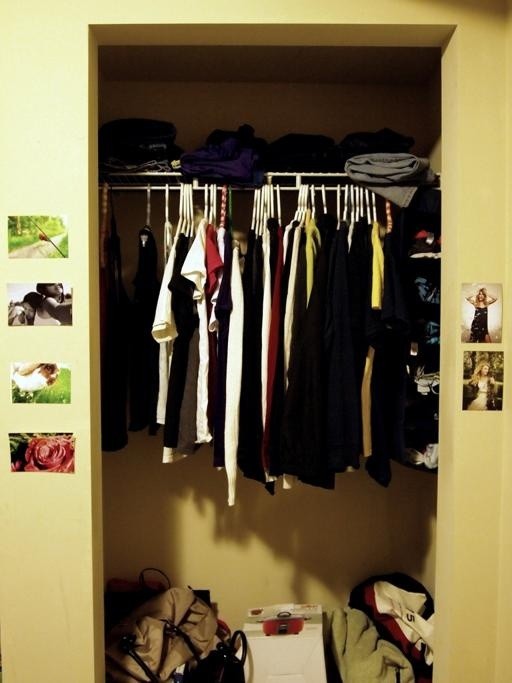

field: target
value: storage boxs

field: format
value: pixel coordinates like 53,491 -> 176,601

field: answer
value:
239,601 -> 329,681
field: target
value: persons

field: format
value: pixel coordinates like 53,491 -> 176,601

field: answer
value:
11,362 -> 61,392
465,361 -> 496,411
8,282 -> 72,326
464,286 -> 498,343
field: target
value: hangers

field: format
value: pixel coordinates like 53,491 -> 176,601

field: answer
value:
97,182 -> 380,252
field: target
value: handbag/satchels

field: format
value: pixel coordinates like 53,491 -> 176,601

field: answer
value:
105,568 -> 247,682
